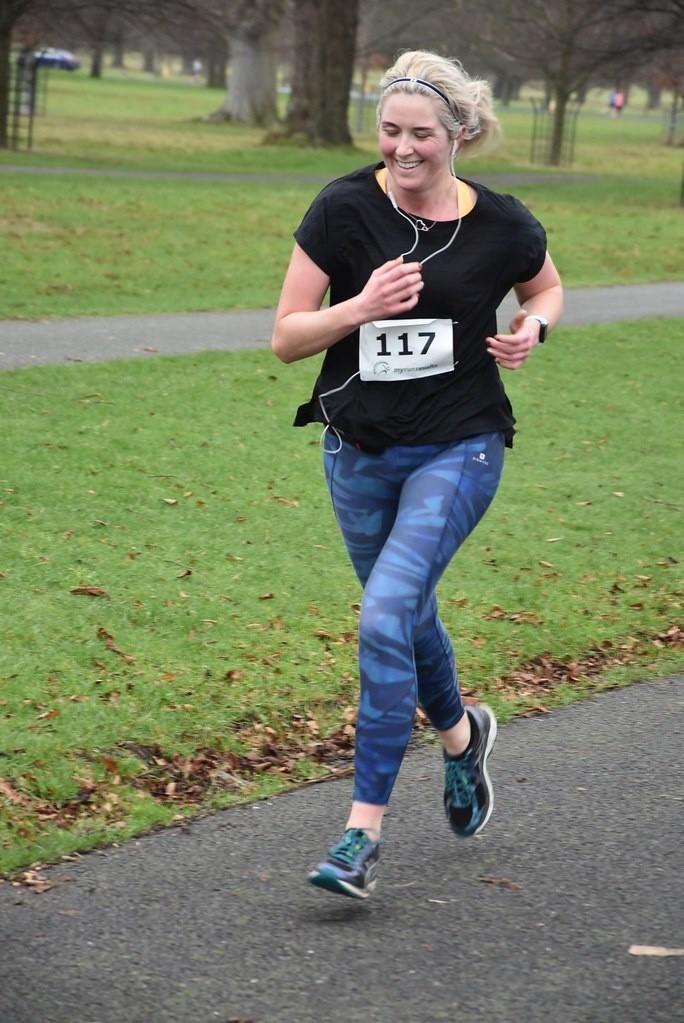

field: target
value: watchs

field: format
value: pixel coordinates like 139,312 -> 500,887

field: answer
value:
525,315 -> 549,344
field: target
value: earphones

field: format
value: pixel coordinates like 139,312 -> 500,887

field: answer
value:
452,140 -> 457,154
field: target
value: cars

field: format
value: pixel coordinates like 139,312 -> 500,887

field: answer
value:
14,45 -> 83,71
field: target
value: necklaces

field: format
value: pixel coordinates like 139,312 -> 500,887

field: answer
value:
390,176 -> 455,231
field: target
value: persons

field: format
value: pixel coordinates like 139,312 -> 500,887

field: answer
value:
607,90 -> 624,119
271,52 -> 563,900
192,57 -> 202,84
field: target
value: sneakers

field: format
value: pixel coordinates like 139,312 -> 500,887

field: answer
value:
442,704 -> 497,838
308,826 -> 380,898
